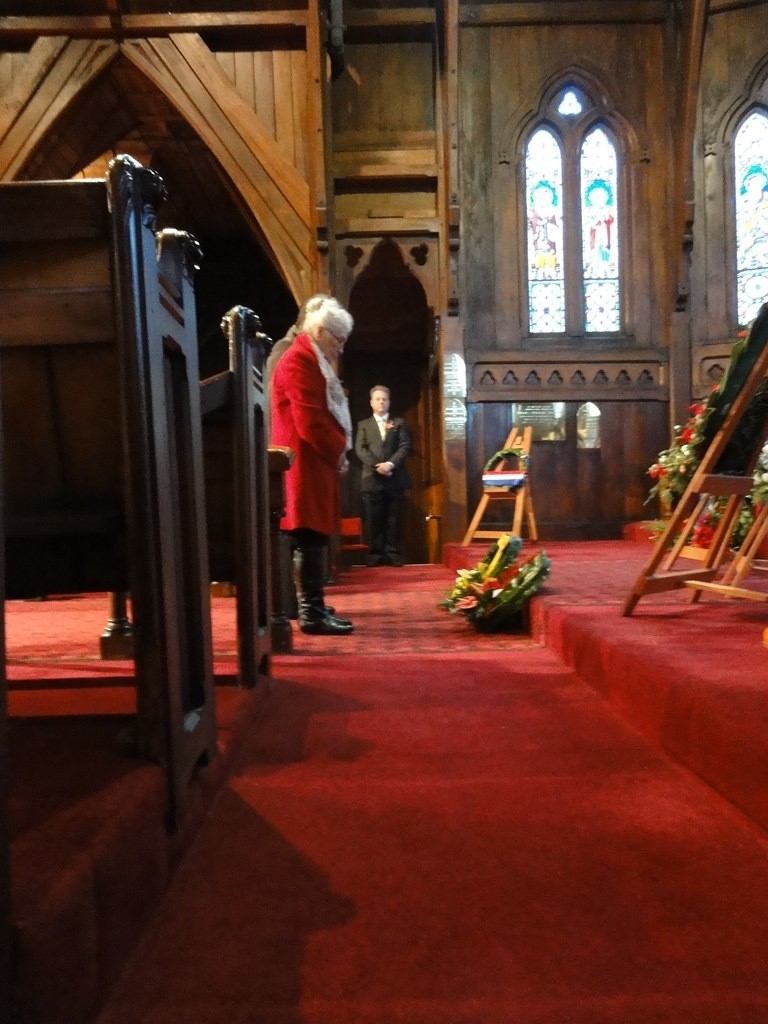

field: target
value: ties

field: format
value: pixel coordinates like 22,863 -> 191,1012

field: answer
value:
379,418 -> 387,441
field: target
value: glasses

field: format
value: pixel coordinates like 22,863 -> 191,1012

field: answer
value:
328,329 -> 345,343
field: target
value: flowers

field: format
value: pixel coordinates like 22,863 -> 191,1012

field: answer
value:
436,564 -> 504,624
640,328 -> 768,562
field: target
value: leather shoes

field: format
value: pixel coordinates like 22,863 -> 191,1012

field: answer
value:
327,580 -> 335,584
300,617 -> 353,634
392,560 -> 401,565
323,606 -> 335,613
367,559 -> 384,566
323,613 -> 351,625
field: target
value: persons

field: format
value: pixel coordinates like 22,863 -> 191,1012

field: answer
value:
265,294 -> 355,635
356,386 -> 414,568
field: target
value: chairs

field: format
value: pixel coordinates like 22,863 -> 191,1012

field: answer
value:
98,447 -> 296,661
336,518 -> 368,568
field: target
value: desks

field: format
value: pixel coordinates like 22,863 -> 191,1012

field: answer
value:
199,304 -> 273,686
0,153 -> 217,836
